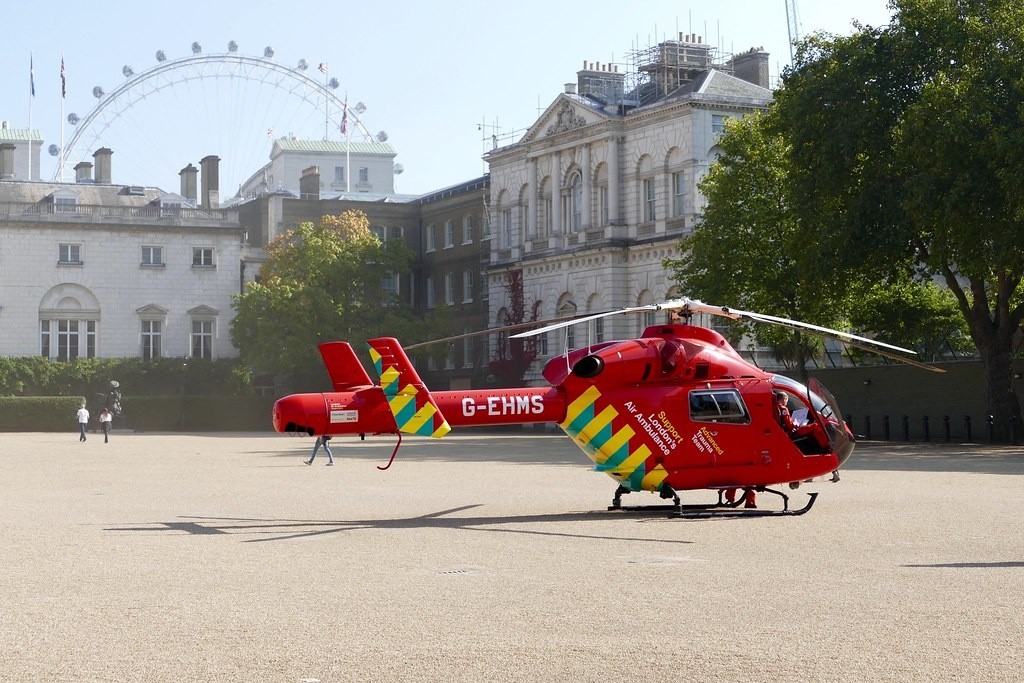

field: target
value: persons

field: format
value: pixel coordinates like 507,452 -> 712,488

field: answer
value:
107,381 -> 122,420
99,408 -> 112,443
777,392 -> 830,454
303,436 -> 334,466
725,489 -> 757,508
76,404 -> 89,442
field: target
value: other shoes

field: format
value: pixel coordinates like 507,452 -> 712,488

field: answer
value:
303,461 -> 311,466
326,463 -> 334,466
745,504 -> 756,508
823,447 -> 829,453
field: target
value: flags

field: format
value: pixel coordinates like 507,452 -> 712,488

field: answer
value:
340,92 -> 349,134
30,51 -> 35,97
60,52 -> 66,98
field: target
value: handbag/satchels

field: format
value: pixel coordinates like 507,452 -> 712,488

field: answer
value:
323,436 -> 332,440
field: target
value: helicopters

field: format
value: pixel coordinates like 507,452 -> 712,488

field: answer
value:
271,296 -> 948,518
